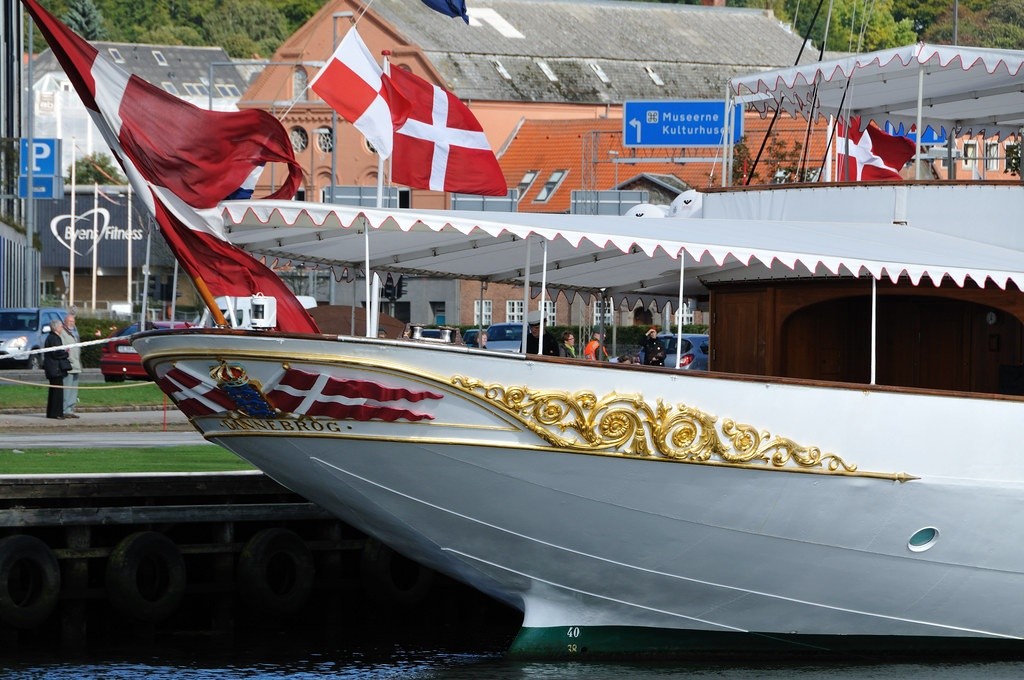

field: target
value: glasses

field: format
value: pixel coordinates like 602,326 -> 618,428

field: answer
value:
568,337 -> 575,341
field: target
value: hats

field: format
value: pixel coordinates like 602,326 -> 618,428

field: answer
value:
592,325 -> 606,334
527,311 -> 547,325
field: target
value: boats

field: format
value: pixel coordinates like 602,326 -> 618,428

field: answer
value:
130,0 -> 1024,664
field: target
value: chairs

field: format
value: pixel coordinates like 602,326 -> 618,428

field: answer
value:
494,328 -> 506,337
13,319 -> 37,330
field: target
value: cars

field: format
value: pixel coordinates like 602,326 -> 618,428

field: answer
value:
422,322 -> 534,353
639,334 -> 709,371
99,321 -> 210,383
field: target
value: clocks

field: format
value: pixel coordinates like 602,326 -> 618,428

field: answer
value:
986,309 -> 1000,326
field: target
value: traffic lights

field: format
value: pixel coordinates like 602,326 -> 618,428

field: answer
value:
385,272 -> 408,301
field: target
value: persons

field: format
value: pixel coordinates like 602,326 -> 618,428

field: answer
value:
470,330 -> 489,349
638,324 -> 667,367
519,311 -> 610,362
110,323 -> 118,331
95,330 -> 101,336
43,315 -> 82,420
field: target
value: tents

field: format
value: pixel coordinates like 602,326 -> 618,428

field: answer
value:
210,198 -> 1024,385
722,43 -> 1024,186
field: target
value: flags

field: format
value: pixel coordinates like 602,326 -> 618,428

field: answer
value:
837,115 -> 916,182
309,26 -> 414,161
389,61 -> 508,197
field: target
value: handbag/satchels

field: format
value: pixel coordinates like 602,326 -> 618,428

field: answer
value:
59,359 -> 72,371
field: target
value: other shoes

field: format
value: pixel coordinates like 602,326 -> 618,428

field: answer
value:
57,414 -> 66,420
64,414 -> 79,418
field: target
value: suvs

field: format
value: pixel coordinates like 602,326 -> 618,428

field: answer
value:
0,309 -> 81,370
